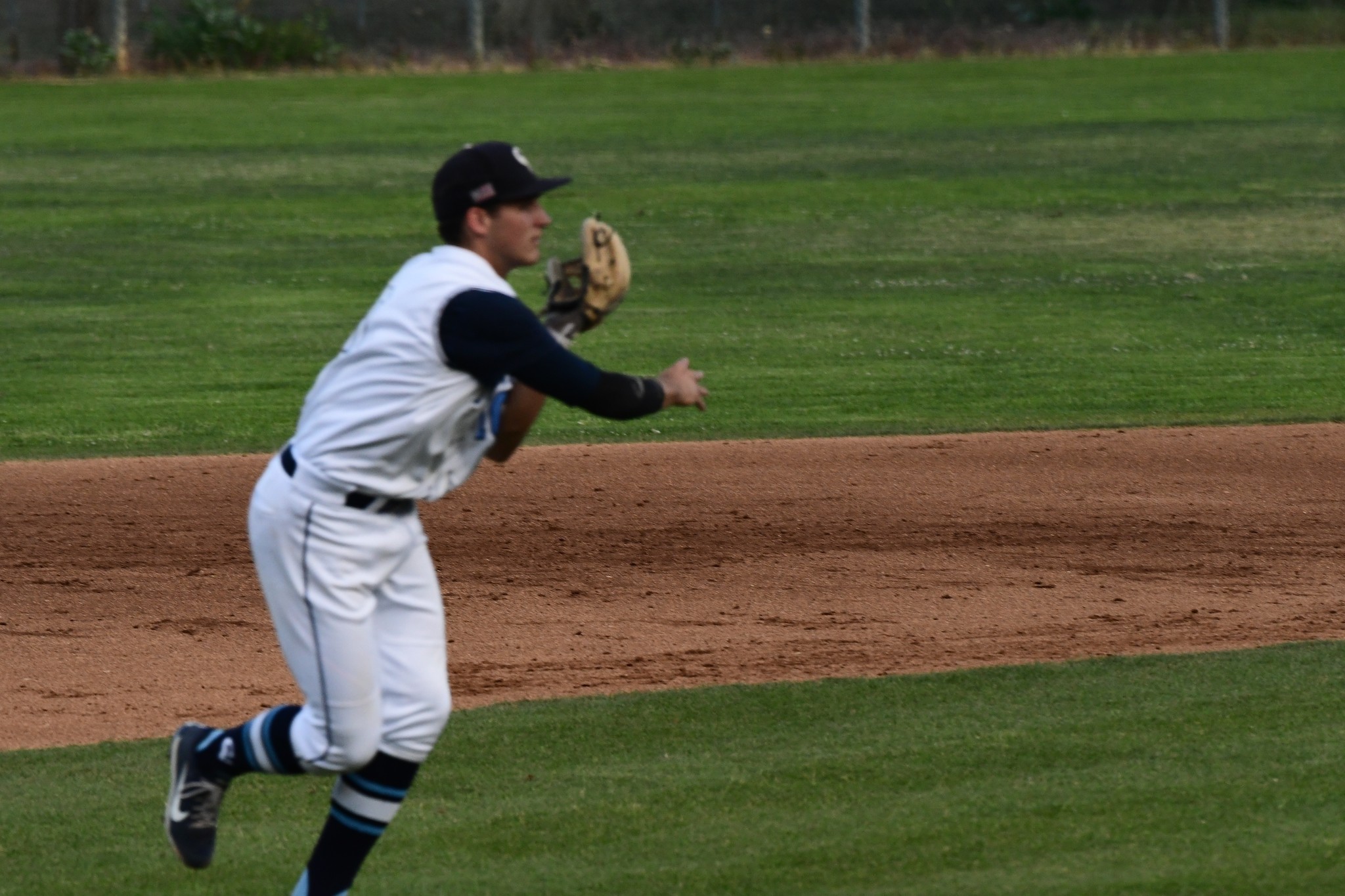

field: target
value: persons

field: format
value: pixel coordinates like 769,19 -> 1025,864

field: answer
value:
166,140 -> 709,896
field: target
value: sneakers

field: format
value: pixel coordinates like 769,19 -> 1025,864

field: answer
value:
164,721 -> 237,870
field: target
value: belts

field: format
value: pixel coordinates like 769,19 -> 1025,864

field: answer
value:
279,446 -> 414,514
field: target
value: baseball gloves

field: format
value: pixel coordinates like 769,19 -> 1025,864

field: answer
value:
546,216 -> 632,341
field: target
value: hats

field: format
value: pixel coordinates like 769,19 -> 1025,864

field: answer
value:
431,139 -> 572,220
